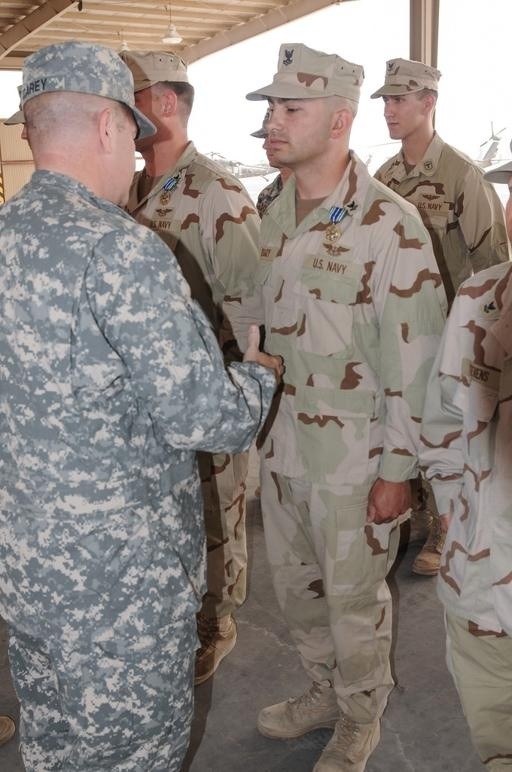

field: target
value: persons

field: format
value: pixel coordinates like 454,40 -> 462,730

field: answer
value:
416,149 -> 512,772
368,57 -> 506,580
249,107 -> 284,220
118,46 -> 262,690
0,41 -> 290,772
216,42 -> 451,772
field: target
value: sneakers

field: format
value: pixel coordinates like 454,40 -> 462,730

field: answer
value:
255,679 -> 342,740
400,509 -> 447,576
312,714 -> 382,772
194,614 -> 238,686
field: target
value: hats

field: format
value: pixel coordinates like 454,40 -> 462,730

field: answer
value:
370,57 -> 442,99
19,40 -> 159,141
245,42 -> 366,105
119,48 -> 190,93
